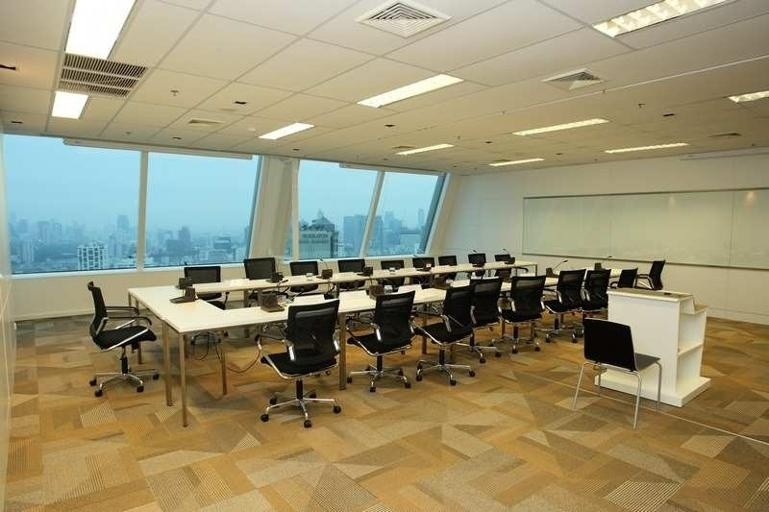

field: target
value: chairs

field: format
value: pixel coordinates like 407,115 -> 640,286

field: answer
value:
494,253 -> 514,280
86,282 -> 162,397
255,301 -> 344,428
412,255 -> 458,288
344,291 -> 415,392
242,257 -> 288,332
412,281 -> 477,385
608,260 -> 665,292
183,266 -> 231,342
500,275 -> 547,354
337,258 -> 367,291
544,262 -> 612,343
573,318 -> 664,430
288,261 -> 321,295
468,253 -> 487,279
380,260 -> 406,287
452,277 -> 504,364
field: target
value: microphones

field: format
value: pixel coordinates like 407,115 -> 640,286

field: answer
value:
599,256 -> 612,269
182,261 -> 198,300
415,245 -> 515,290
546,259 -> 569,277
260,257 -> 398,313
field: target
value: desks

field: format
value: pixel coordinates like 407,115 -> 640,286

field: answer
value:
545,269 -> 622,323
318,288 -> 453,390
336,261 -> 537,318
450,277 -> 533,340
127,284 -> 227,407
163,295 -> 344,427
193,272 -> 339,338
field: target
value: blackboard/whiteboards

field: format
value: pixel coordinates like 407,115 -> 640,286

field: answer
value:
521,186 -> 769,270
